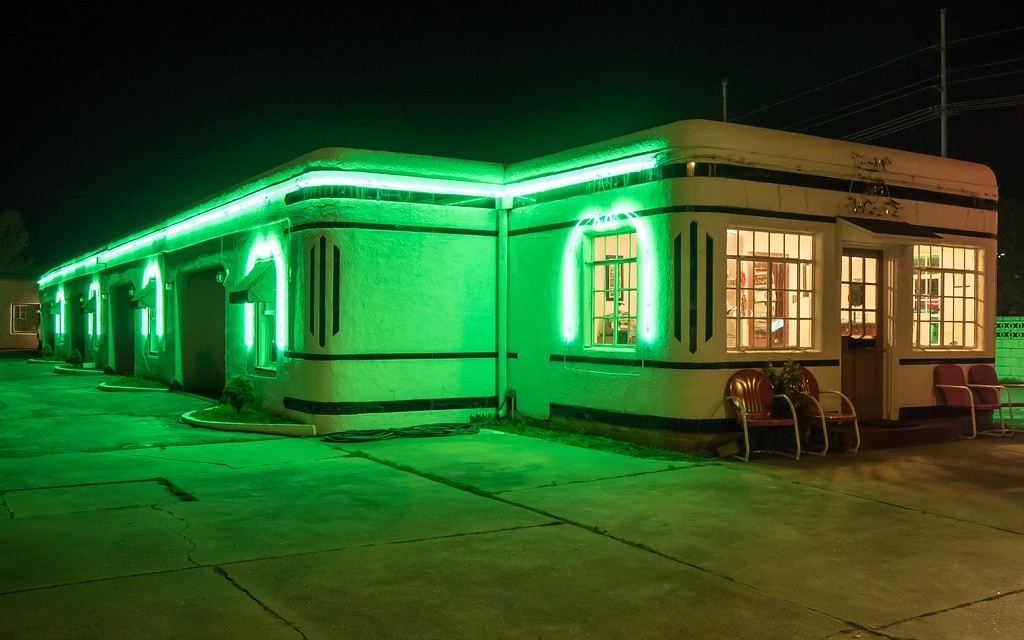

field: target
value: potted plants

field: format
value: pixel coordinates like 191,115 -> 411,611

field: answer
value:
768,356 -> 810,449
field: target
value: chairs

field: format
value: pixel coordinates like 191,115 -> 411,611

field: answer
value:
726,369 -> 801,460
934,364 -> 1024,439
792,366 -> 860,458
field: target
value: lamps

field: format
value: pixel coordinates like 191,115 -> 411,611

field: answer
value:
101,293 -> 107,300
165,280 -> 175,291
129,287 -> 135,297
216,269 -> 226,283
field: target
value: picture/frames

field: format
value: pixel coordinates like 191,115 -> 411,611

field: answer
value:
606,255 -> 624,300
